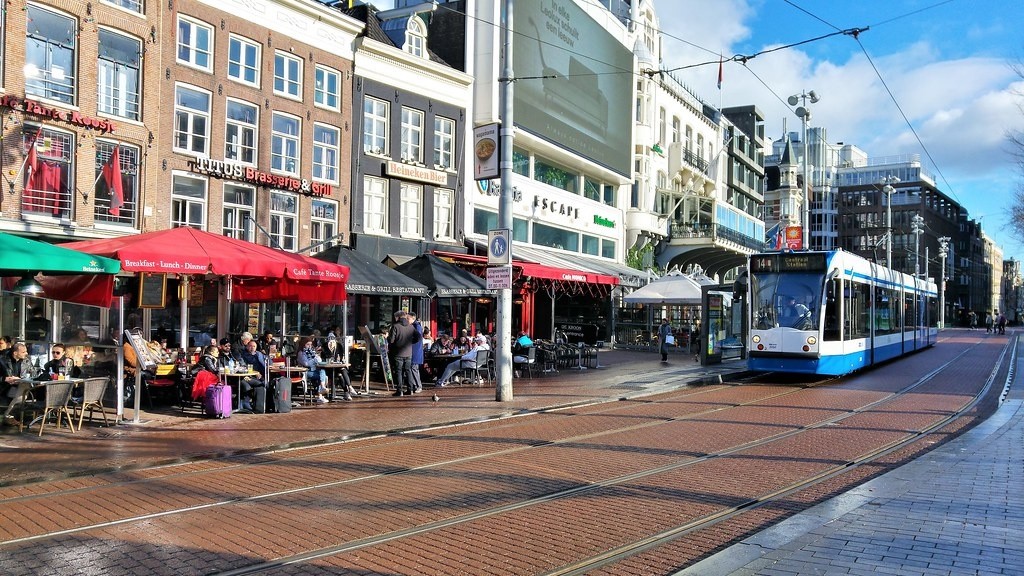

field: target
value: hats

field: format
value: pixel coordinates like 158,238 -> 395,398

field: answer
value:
326,333 -> 337,342
301,338 -> 311,346
475,335 -> 487,342
220,339 -> 229,346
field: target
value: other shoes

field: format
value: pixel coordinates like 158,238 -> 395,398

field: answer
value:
244,403 -> 252,410
349,388 -> 357,396
404,390 -> 413,395
343,392 -> 352,402
317,397 -> 330,404
514,370 -> 522,378
320,388 -> 329,394
435,374 -> 484,388
661,358 -> 668,362
415,387 -> 422,393
392,389 -> 403,396
2,417 -> 20,427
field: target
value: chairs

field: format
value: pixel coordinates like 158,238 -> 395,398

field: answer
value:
459,348 -> 538,387
19,378 -> 111,436
143,363 -> 203,415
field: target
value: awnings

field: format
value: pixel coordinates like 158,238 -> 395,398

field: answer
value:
466,238 -> 658,334
433,250 -> 619,339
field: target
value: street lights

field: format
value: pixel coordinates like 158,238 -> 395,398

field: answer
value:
936,236 -> 952,328
880,174 -> 901,269
910,213 -> 924,279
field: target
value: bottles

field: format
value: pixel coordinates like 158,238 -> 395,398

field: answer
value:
228,358 -> 234,374
281,346 -> 285,358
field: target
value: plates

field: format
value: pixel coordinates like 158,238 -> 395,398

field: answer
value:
318,362 -> 327,364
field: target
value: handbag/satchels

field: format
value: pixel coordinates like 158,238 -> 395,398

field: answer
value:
665,334 -> 675,345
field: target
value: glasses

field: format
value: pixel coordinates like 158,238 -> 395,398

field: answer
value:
52,351 -> 64,355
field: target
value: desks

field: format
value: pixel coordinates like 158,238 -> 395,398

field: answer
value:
435,354 -> 466,383
220,362 -> 352,419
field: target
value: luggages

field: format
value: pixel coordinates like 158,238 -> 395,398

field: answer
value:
270,355 -> 293,413
205,357 -> 232,418
252,358 -> 269,413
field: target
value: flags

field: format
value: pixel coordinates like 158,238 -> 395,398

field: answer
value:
708,155 -> 719,180
28,127 -> 41,182
768,224 -> 782,250
102,147 -> 123,216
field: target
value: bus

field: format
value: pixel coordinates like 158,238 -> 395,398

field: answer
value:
733,247 -> 939,376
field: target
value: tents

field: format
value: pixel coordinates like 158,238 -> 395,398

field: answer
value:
393,246 -> 498,299
0,229 -> 120,274
623,267 -> 714,304
52,224 -> 350,360
307,244 -> 428,364
691,272 -> 741,307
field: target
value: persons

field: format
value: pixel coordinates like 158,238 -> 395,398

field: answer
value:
123,326 -> 358,414
422,325 -> 533,387
969,311 -> 1006,335
0,307 -> 119,427
378,311 -> 424,397
684,322 -> 701,362
657,318 -> 671,362
784,297 -> 811,317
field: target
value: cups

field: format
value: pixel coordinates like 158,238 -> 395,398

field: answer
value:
276,351 -> 281,357
461,346 -> 464,355
248,365 -> 253,374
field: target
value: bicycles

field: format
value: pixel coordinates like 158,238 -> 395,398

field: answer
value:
554,326 -> 568,344
